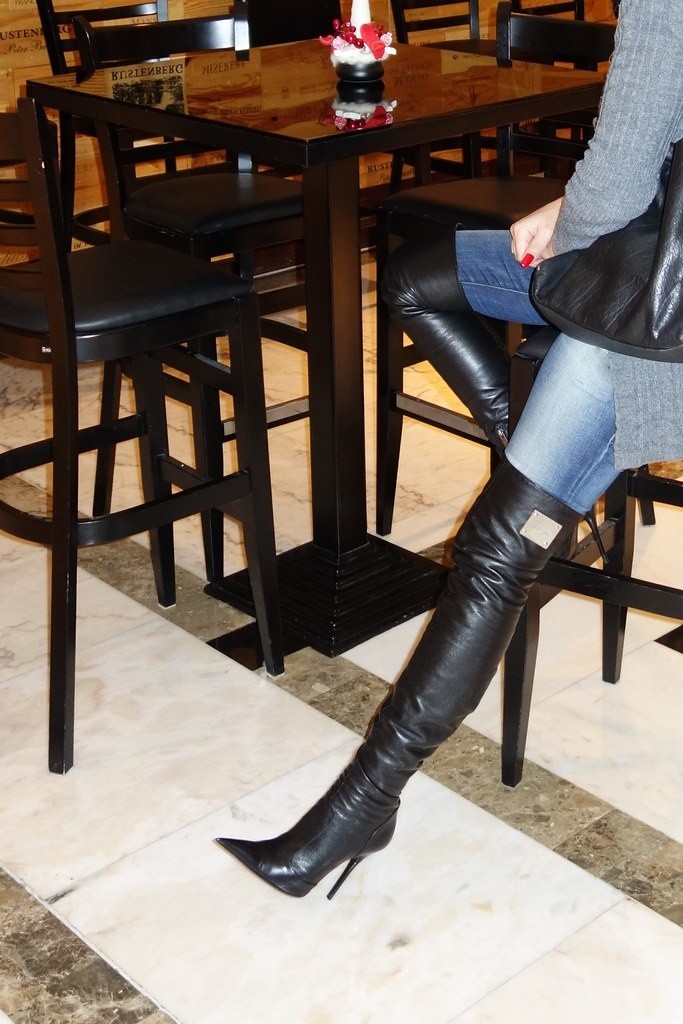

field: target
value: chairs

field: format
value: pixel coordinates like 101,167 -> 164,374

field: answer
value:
36,0 -> 374,581
229,0 -> 344,48
377,2 -> 617,539
1,96 -> 287,777
513,0 -> 585,20
510,0 -> 602,157
375,0 -> 571,536
502,355 -> 683,791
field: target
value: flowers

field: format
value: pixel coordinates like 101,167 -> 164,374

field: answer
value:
319,19 -> 397,61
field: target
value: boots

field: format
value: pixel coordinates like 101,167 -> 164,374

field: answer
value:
214,449 -> 585,901
378,220 -> 609,564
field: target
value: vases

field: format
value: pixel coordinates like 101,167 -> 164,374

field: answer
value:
336,62 -> 386,104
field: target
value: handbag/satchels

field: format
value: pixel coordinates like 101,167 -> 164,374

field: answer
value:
528,140 -> 683,364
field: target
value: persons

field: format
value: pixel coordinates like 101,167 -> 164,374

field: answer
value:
215,0 -> 683,900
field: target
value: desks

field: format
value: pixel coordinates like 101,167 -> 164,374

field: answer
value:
24,35 -> 610,657
